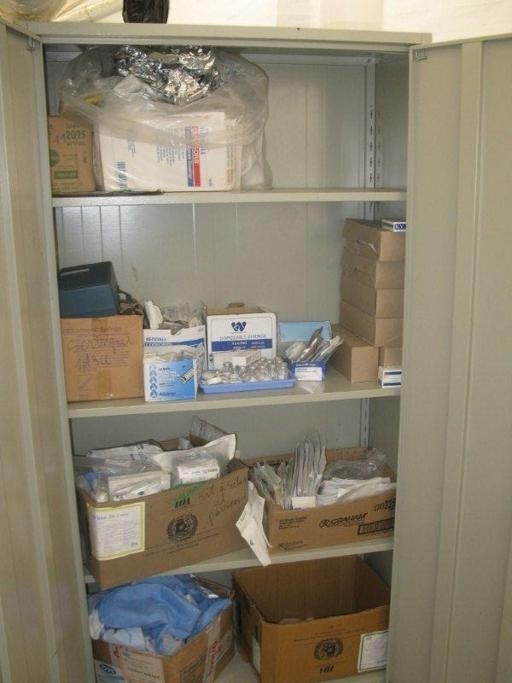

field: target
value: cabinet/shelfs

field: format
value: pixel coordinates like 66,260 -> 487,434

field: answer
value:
1,18 -> 512,683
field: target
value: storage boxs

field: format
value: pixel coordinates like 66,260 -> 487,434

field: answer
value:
232,554 -> 390,683
75,434 -> 250,593
249,448 -> 397,555
61,313 -> 144,402
91,577 -> 236,683
94,112 -> 239,193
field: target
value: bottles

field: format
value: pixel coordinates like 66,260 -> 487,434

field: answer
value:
218,355 -> 289,383
